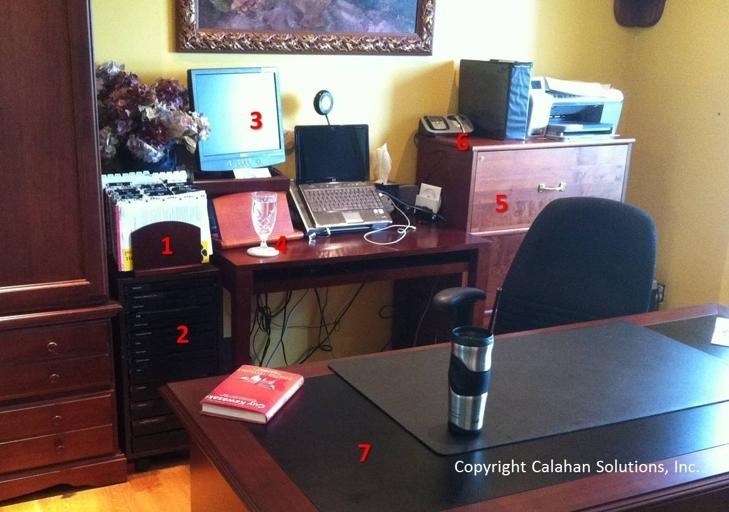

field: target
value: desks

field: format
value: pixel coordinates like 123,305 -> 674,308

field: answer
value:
212,220 -> 494,377
155,298 -> 729,512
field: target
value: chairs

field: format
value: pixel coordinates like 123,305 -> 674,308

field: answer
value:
404,194 -> 664,351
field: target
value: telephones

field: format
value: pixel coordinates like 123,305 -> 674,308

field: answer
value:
419,114 -> 474,138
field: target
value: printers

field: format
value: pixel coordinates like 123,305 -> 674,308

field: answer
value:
526,77 -> 623,141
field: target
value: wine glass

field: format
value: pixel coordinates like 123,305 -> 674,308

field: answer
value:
246,191 -> 281,258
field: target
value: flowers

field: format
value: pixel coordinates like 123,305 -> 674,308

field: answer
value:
93,58 -> 214,164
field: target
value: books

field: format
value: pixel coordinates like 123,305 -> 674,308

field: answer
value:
199,361 -> 306,425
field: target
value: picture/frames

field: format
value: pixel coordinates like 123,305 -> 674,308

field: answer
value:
173,0 -> 441,60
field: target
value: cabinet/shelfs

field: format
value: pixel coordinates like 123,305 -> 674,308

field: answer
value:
0,304 -> 134,504
0,0 -> 114,319
391,133 -> 635,350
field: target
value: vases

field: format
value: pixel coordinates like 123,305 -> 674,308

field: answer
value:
115,117 -> 179,173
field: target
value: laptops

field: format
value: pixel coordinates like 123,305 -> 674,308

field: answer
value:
293,125 -> 393,227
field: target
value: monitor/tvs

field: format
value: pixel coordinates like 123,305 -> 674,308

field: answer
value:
186,67 -> 286,172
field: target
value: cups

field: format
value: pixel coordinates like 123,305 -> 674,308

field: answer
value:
449,325 -> 494,440
